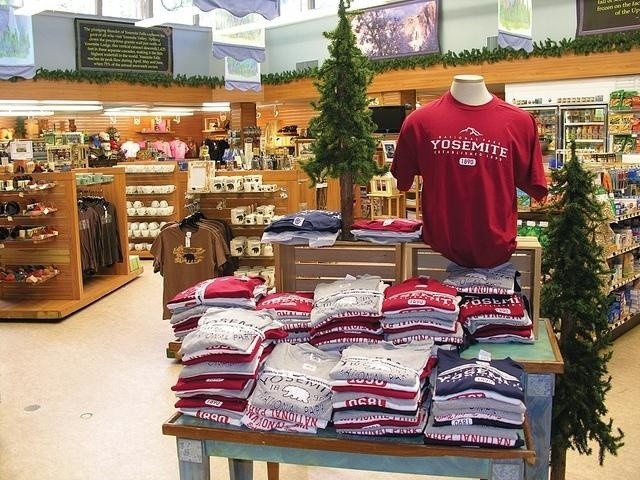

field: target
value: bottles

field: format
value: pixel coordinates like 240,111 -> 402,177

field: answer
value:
517,220 -> 549,274
565,126 -> 603,140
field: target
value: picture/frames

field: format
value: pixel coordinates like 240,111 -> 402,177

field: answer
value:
342,1 -> 443,58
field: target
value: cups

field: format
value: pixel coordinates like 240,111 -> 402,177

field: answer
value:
76,172 -> 114,185
126,184 -> 176,250
230,203 -> 275,288
212,175 -> 263,192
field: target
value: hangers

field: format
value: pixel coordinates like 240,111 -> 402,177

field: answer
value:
76,185 -> 109,208
176,196 -> 208,234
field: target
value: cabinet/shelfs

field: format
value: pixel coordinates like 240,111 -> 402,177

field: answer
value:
0,179 -> 65,295
115,162 -> 181,260
575,154 -> 640,347
174,166 -> 295,302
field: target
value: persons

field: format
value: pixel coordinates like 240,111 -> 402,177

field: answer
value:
120,137 -> 190,160
389,74 -> 548,268
